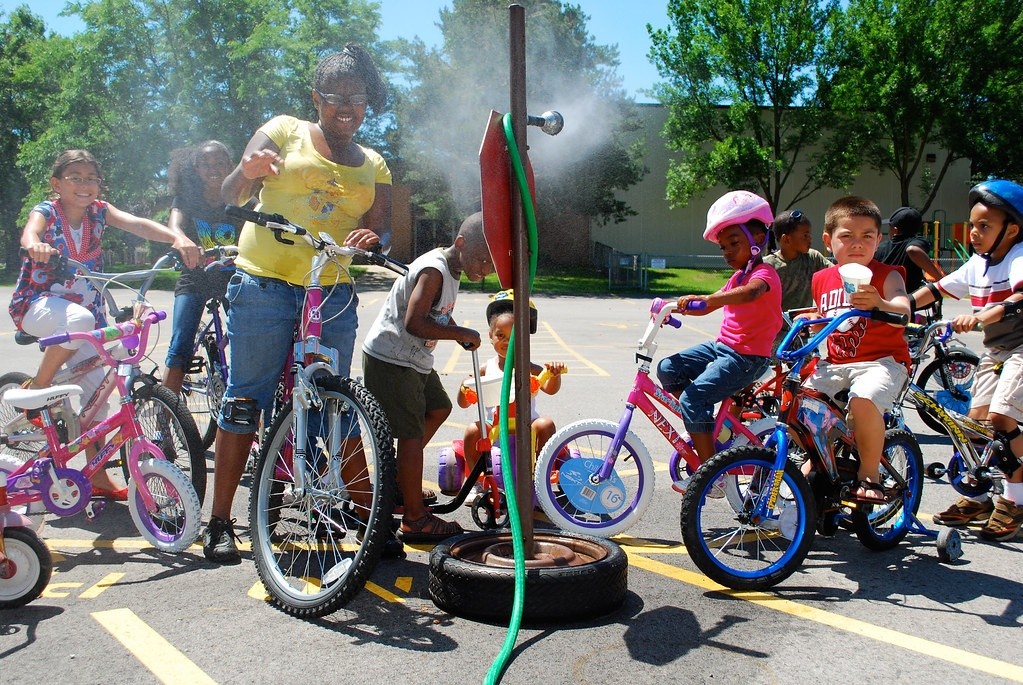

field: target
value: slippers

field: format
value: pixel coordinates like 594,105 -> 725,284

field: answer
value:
856,480 -> 887,505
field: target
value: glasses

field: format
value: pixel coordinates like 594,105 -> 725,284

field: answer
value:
60,174 -> 103,186
783,210 -> 805,235
315,89 -> 370,108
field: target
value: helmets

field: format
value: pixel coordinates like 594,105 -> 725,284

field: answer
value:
968,180 -> 1023,227
486,289 -> 537,334
703,190 -> 779,243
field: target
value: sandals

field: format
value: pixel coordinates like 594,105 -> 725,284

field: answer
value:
394,487 -> 438,507
396,510 -> 464,543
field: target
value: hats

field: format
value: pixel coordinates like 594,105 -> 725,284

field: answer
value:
882,207 -> 922,234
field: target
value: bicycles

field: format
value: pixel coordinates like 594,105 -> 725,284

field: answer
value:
0,203 -> 411,623
532,296 -> 1023,591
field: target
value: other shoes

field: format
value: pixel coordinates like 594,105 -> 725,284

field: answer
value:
24,408 -> 44,428
464,483 -> 484,505
90,486 -> 130,500
342,505 -> 359,528
781,447 -> 815,500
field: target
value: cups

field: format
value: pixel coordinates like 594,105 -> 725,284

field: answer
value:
839,263 -> 872,302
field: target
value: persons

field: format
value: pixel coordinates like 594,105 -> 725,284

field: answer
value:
656,190 -> 783,500
873,206 -> 942,296
910,179 -> 1023,541
362,212 -> 494,543
458,286 -> 564,506
762,211 -> 835,397
201,42 -> 403,561
9,149 -> 198,500
153,139 -> 259,464
792,197 -> 912,508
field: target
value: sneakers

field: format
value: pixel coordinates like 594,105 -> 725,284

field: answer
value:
354,520 -> 404,553
314,508 -> 347,539
932,494 -> 995,526
980,493 -> 1022,542
202,515 -> 243,564
671,472 -> 726,499
